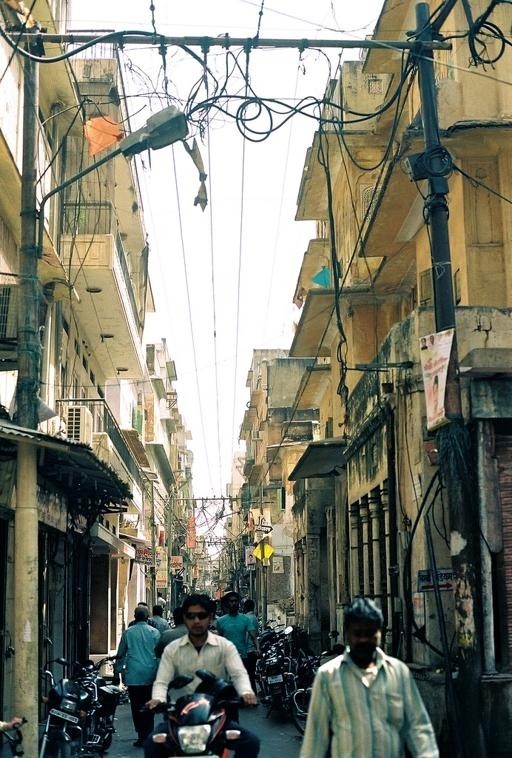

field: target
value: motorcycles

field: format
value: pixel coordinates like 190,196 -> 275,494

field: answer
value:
138,669 -> 260,757
255,618 -> 345,736
39,654 -> 122,757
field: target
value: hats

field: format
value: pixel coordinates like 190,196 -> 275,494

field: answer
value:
342,596 -> 386,630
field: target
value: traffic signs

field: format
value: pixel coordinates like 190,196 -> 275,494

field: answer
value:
418,568 -> 454,590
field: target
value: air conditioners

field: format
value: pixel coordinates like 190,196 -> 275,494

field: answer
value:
68,405 -> 93,447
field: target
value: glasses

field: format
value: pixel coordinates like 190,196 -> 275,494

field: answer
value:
185,611 -> 211,620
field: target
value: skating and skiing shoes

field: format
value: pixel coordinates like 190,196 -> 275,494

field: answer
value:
132,738 -> 145,748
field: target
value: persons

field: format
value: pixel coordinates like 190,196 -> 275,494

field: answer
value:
420,338 -> 428,351
0,715 -> 22,732
432,376 -> 439,414
296,596 -> 442,757
113,588 -> 262,757
248,553 -> 254,563
429,335 -> 434,346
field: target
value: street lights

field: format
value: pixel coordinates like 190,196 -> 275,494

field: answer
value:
13,50 -> 190,756
